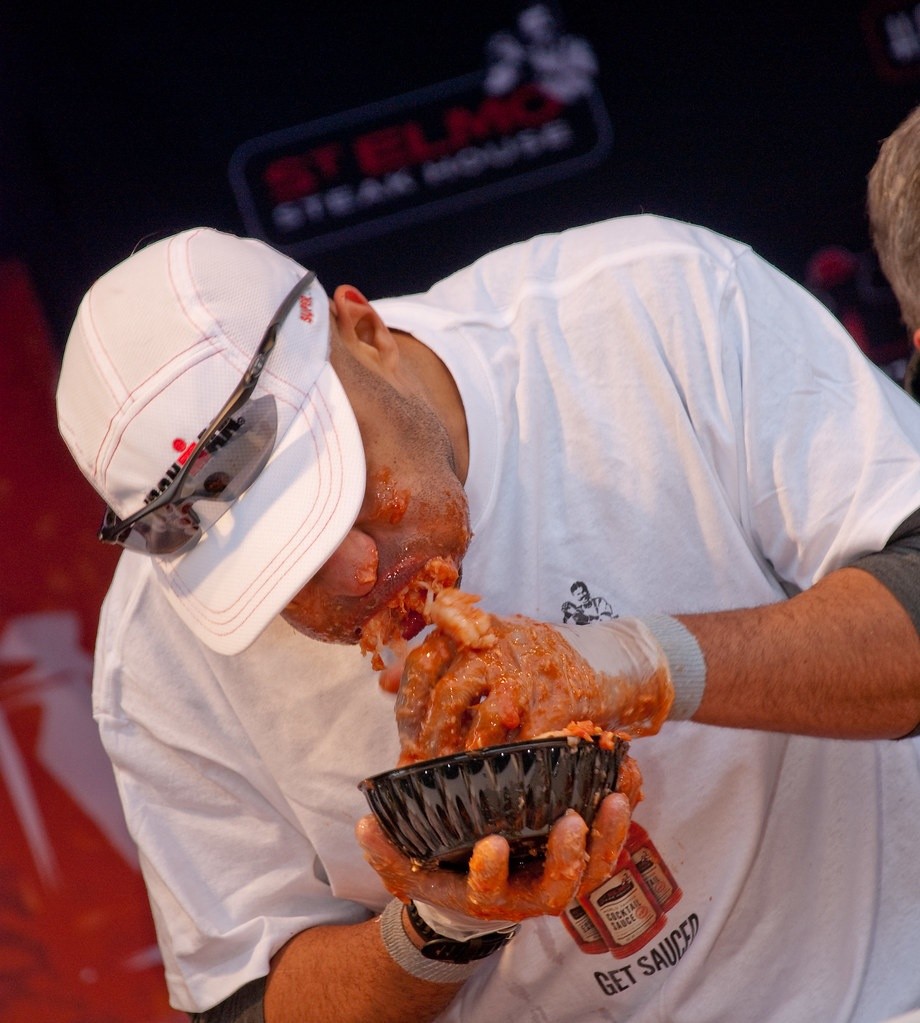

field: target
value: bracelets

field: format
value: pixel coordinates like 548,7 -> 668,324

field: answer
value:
406,901 -> 525,960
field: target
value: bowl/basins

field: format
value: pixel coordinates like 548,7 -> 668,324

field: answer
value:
356,731 -> 630,877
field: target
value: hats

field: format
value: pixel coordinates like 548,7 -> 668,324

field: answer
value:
53,227 -> 366,659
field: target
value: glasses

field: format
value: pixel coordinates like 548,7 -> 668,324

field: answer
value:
99,269 -> 317,559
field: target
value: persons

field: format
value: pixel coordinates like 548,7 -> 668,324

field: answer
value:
52,217 -> 920,1023
866,109 -> 920,357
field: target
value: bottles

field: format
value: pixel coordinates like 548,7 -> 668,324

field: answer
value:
557,816 -> 682,962
